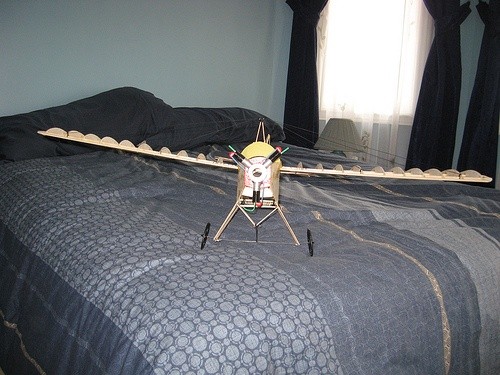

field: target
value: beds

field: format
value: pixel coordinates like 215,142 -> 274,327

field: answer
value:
0,142 -> 500,375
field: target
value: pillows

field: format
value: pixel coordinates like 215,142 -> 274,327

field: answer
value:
0,86 -> 174,164
146,107 -> 286,152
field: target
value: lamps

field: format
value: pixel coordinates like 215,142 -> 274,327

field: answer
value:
313,118 -> 364,159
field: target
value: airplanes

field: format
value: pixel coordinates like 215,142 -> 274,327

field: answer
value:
35,126 -> 491,257
